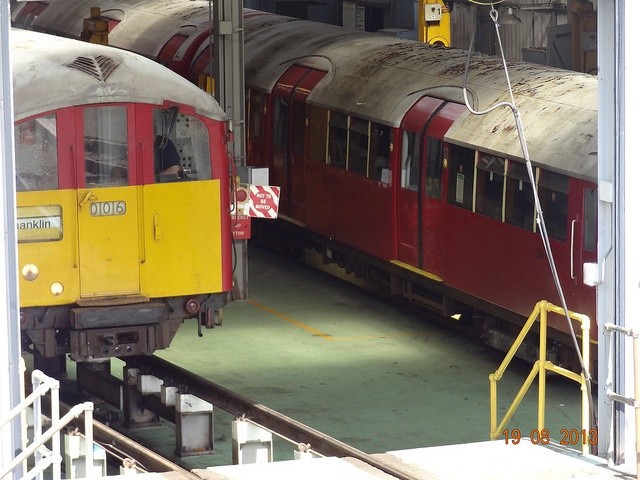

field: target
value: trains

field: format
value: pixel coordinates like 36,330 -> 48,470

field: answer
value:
10,0 -> 598,385
9,26 -> 233,364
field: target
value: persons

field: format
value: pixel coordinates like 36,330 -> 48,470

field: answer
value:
111,122 -> 181,181
